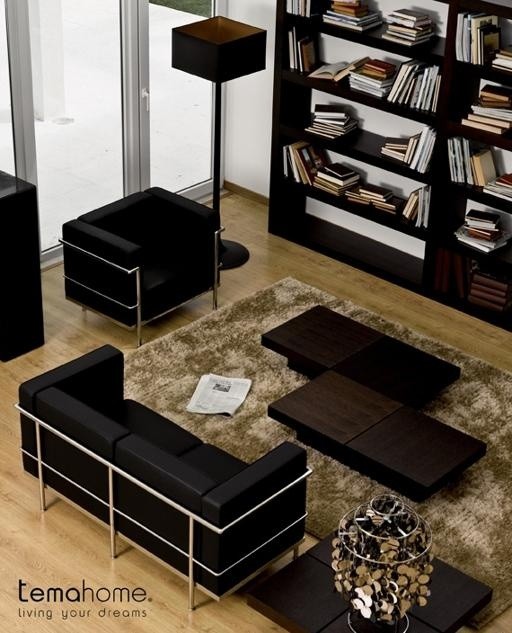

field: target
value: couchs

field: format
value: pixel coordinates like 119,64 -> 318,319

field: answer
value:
12,345 -> 312,613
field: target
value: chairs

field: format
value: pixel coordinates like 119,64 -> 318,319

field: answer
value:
58,186 -> 225,345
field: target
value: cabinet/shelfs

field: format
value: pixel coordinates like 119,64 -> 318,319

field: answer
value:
267,0 -> 511,330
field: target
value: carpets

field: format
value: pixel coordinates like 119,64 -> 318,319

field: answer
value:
122,277 -> 511,631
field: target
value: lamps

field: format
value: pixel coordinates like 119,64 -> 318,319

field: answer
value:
169,14 -> 269,271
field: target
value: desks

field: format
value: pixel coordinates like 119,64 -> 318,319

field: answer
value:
245,515 -> 494,633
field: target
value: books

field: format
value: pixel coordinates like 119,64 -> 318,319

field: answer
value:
183,373 -> 252,419
282,0 -> 512,312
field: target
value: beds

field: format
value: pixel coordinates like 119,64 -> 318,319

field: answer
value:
254,304 -> 489,504
